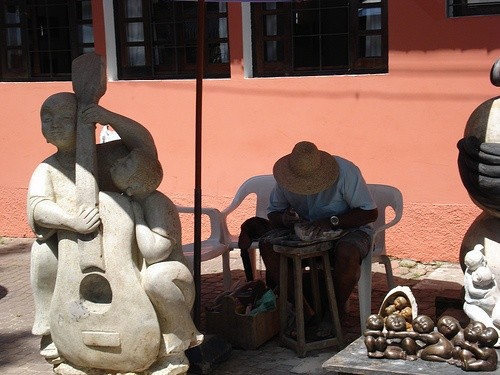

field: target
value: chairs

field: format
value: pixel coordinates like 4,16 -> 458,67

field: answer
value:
358,184 -> 403,335
218,173 -> 277,289
175,205 -> 228,276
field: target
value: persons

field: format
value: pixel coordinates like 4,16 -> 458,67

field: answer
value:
22,92 -> 205,358
259,141 -> 378,337
364,297 -> 498,372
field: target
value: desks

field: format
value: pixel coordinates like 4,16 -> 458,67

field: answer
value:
322,325 -> 500,375
272,241 -> 345,359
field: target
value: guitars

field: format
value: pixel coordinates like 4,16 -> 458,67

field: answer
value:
49,50 -> 163,371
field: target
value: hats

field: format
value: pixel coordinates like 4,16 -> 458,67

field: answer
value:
273,141 -> 339,196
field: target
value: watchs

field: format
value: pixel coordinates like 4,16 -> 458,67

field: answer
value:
330,215 -> 339,231
